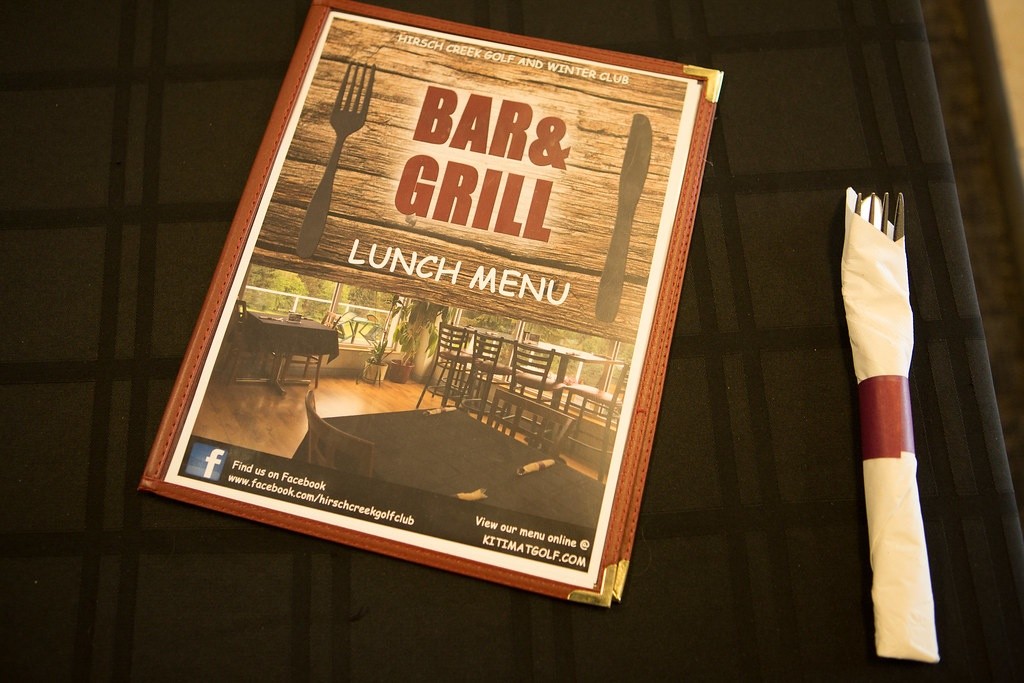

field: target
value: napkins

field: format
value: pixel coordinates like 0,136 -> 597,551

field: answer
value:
839,186 -> 941,664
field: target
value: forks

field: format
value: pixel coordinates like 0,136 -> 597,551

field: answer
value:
855,193 -> 904,242
595,113 -> 653,322
297,60 -> 376,260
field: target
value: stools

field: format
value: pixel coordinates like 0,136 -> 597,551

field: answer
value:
457,330 -> 516,421
561,363 -> 631,483
494,340 -> 565,450
416,321 -> 474,408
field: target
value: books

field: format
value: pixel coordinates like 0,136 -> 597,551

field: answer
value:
138,0 -> 723,608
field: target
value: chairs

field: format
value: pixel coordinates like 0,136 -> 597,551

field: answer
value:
280,312 -> 341,388
303,390 -> 375,521
220,300 -> 262,385
487,390 -> 577,458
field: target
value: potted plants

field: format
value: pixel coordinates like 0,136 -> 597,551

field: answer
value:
355,292 -> 409,389
385,295 -> 451,384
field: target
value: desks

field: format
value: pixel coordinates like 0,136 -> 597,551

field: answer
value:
233,311 -> 340,398
463,325 -> 611,467
276,406 -> 605,573
